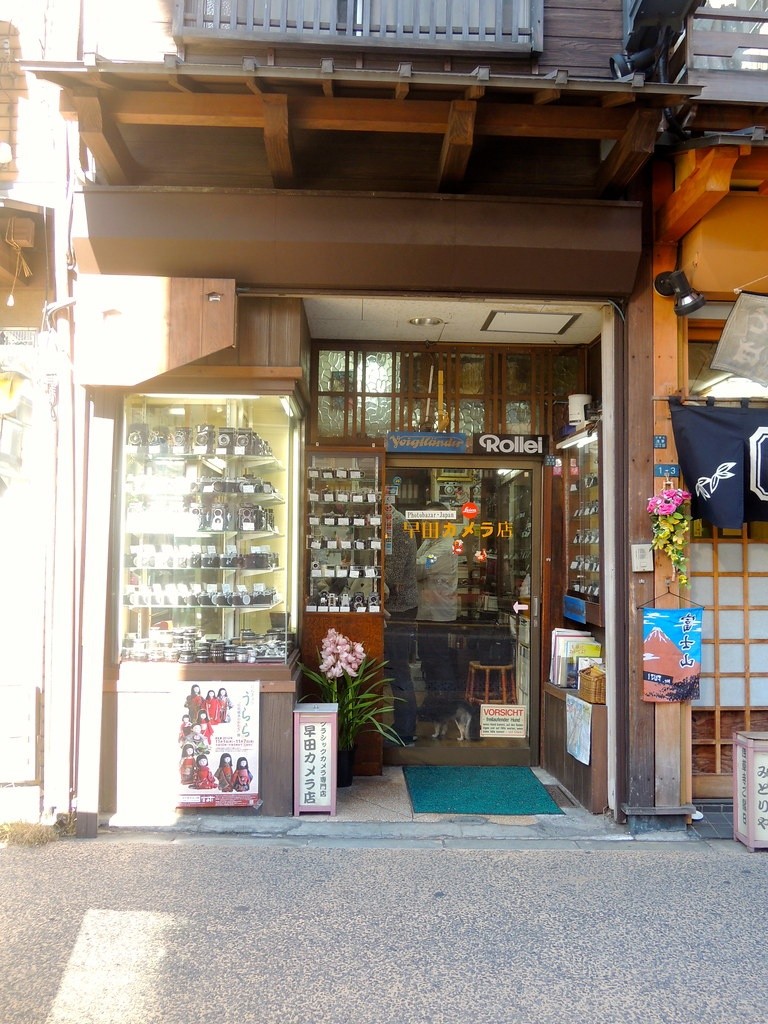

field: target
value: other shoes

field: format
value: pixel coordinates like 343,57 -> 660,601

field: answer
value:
383,731 -> 417,747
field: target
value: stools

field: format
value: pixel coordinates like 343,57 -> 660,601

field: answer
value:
463,661 -> 518,709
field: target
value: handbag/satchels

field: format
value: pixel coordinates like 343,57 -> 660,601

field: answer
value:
478,618 -> 512,666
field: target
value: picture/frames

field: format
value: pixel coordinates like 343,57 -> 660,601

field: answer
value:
436,469 -> 472,482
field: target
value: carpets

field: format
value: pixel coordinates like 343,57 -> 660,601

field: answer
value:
400,765 -> 564,816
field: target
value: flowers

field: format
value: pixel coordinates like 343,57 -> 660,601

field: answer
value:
293,626 -> 407,751
647,488 -> 694,586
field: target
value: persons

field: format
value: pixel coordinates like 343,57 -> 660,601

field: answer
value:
383,496 -> 421,749
416,501 -> 459,721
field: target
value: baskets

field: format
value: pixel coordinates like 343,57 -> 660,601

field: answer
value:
579,662 -> 606,704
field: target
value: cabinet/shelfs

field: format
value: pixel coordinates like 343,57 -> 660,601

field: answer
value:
104,390 -> 307,680
561,421 -> 605,627
542,682 -> 607,814
306,445 -> 383,617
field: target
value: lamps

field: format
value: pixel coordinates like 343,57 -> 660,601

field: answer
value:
555,427 -> 591,449
609,48 -> 655,80
654,270 -> 708,317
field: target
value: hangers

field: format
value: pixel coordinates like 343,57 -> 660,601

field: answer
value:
636,576 -> 705,611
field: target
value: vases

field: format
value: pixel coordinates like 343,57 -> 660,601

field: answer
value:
335,751 -> 354,787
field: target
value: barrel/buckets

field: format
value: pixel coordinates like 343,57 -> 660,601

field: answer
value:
568,393 -> 592,425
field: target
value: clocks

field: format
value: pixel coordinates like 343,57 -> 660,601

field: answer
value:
444,484 -> 454,494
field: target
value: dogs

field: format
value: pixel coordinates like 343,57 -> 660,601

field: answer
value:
416,696 -> 477,742
